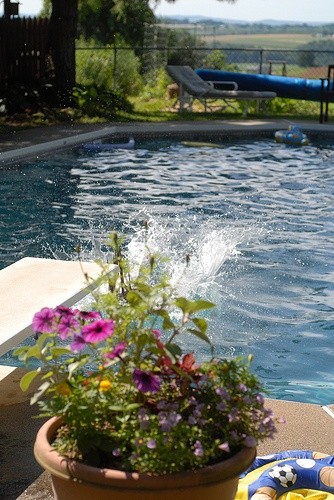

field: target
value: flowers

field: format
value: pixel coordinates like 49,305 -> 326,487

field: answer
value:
14,231 -> 287,475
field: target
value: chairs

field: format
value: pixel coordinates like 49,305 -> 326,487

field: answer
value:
167,62 -> 276,118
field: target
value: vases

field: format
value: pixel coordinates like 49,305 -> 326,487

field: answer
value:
33,416 -> 257,500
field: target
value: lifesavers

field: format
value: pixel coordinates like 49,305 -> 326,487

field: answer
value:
239,449 -> 334,500
78,135 -> 135,150
178,140 -> 225,149
273,124 -> 310,146
